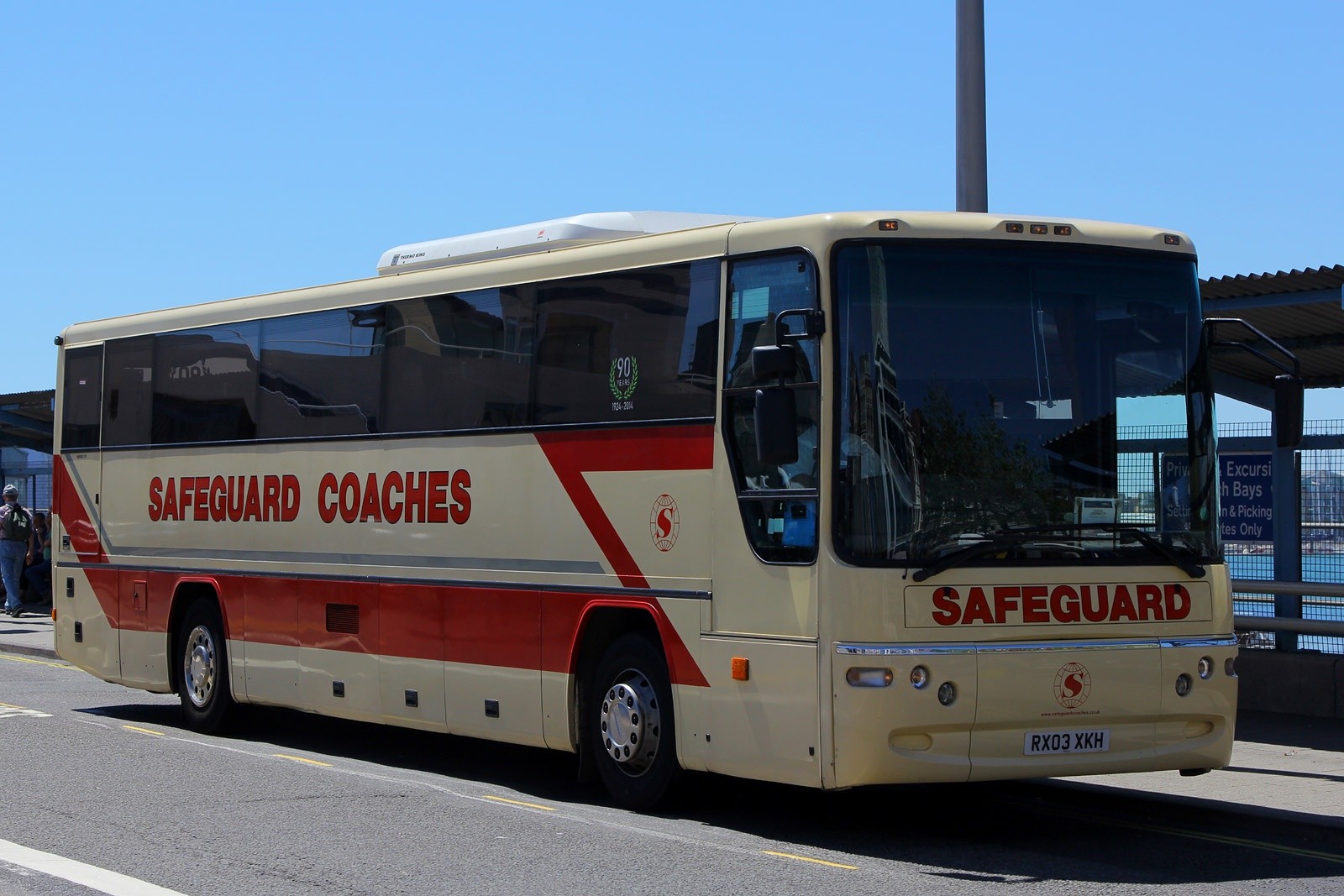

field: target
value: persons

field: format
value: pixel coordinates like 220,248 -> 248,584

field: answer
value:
0,484 -> 52,618
937,408 -> 1052,537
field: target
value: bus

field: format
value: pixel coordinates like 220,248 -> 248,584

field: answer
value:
49,206 -> 1307,817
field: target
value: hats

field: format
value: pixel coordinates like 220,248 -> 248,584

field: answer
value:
3,484 -> 19,496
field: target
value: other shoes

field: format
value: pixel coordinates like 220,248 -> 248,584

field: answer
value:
5,608 -> 12,615
37,590 -> 52,605
10,604 -> 24,618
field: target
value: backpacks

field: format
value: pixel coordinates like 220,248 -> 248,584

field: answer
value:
1,504 -> 31,540
